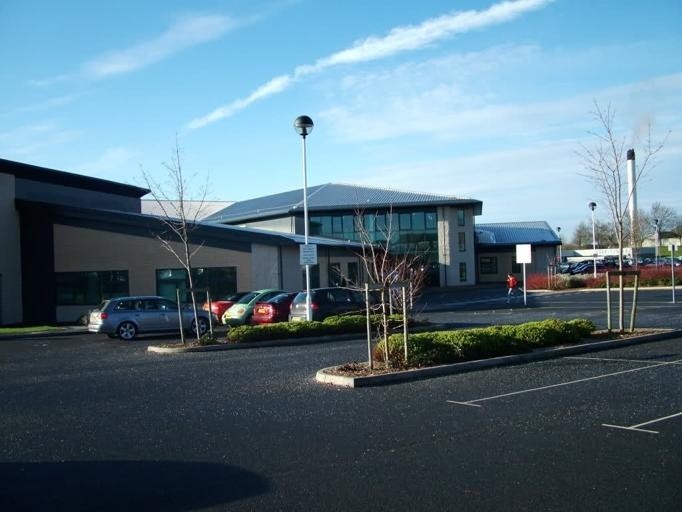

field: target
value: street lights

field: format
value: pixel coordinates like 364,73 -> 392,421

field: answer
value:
651,216 -> 661,266
559,255 -> 682,276
292,115 -> 319,321
587,201 -> 599,278
557,226 -> 563,262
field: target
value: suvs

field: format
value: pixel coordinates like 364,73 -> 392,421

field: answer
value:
86,295 -> 218,338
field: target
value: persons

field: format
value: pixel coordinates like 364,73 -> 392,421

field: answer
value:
506,274 -> 518,296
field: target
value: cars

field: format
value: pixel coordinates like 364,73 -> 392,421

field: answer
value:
203,286 -> 379,324
